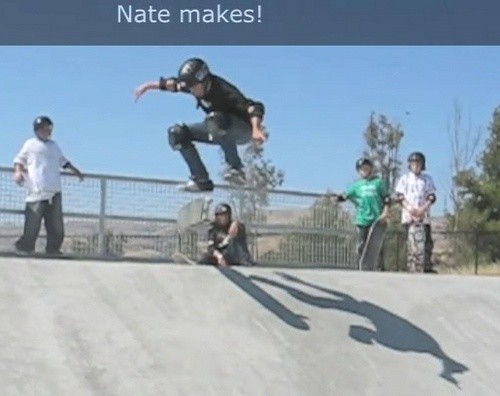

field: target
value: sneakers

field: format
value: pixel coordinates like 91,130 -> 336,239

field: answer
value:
224,168 -> 245,185
176,176 -> 213,192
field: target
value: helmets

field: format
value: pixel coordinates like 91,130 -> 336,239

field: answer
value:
356,158 -> 371,169
215,203 -> 231,215
178,59 -> 211,90
408,152 -> 426,171
34,116 -> 53,131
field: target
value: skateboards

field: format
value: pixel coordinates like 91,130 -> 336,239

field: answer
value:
1,249 -> 69,258
409,224 -> 427,271
174,197 -> 212,233
359,219 -> 387,270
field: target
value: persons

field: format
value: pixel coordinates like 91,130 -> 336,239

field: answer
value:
330,158 -> 391,272
395,151 -> 439,273
197,203 -> 255,267
14,115 -> 84,257
133,58 -> 270,192
